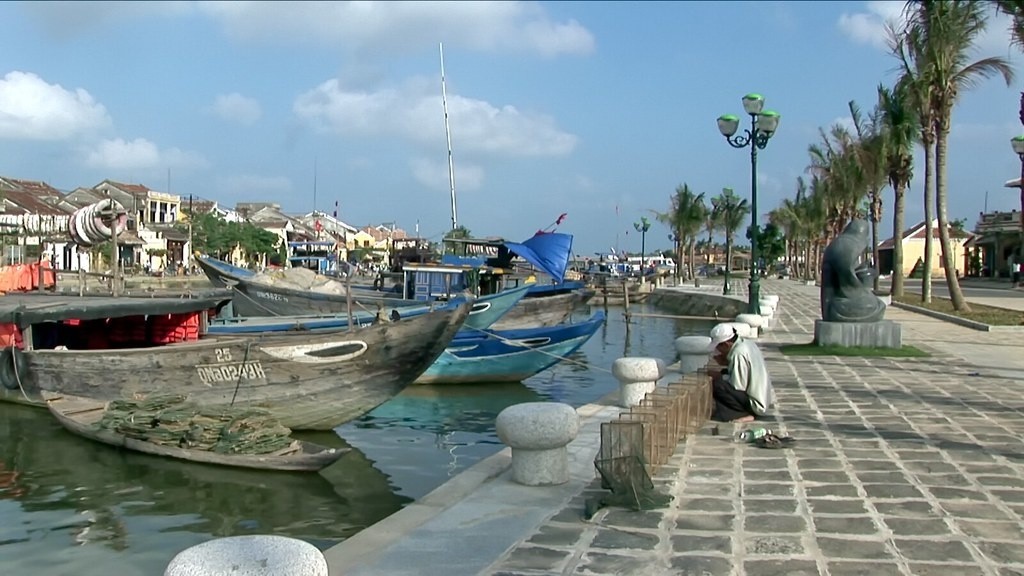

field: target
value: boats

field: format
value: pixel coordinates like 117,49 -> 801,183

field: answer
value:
0,42 -> 655,431
41,389 -> 353,471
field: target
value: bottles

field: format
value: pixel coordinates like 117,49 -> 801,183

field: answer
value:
745,429 -> 771,443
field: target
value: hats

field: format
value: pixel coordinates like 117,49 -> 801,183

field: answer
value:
706,323 -> 735,348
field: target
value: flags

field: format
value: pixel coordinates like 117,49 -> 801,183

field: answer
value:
315,220 -> 323,231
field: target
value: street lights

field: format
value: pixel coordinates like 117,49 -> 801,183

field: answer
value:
716,93 -> 781,313
633,216 -> 651,270
668,229 -> 682,286
711,188 -> 740,295
1011,135 -> 1024,286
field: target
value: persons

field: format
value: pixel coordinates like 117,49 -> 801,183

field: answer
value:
1013,260 -> 1021,288
708,322 -> 770,423
